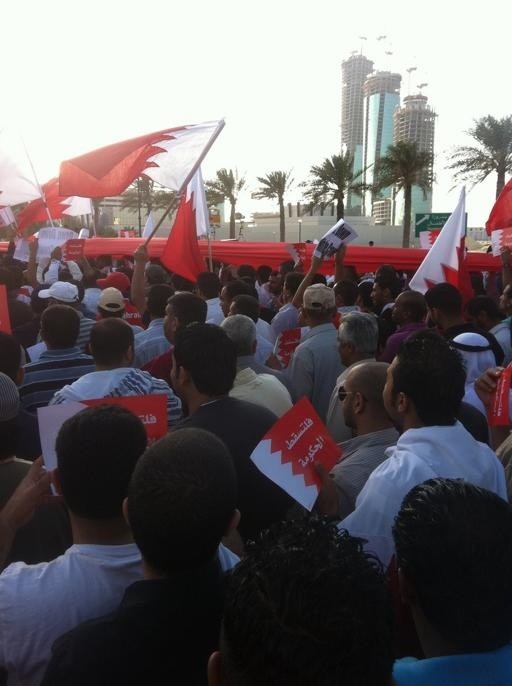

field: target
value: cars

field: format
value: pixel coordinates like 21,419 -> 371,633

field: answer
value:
375,217 -> 386,225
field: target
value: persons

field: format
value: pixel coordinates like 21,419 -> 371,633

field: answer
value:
1,239 -> 511,684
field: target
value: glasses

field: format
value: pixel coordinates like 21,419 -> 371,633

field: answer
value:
337,385 -> 367,402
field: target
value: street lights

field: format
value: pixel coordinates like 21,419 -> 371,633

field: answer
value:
386,197 -> 393,225
298,219 -> 303,243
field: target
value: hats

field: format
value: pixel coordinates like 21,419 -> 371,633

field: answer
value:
37,280 -> 79,303
96,271 -> 130,290
301,283 -> 336,310
96,287 -> 127,313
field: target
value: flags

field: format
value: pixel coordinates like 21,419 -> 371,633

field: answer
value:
486,179 -> 512,236
0,123 -> 227,281
419,230 -> 440,249
409,186 -> 478,301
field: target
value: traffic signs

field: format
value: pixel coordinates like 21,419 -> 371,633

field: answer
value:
414,212 -> 468,239
